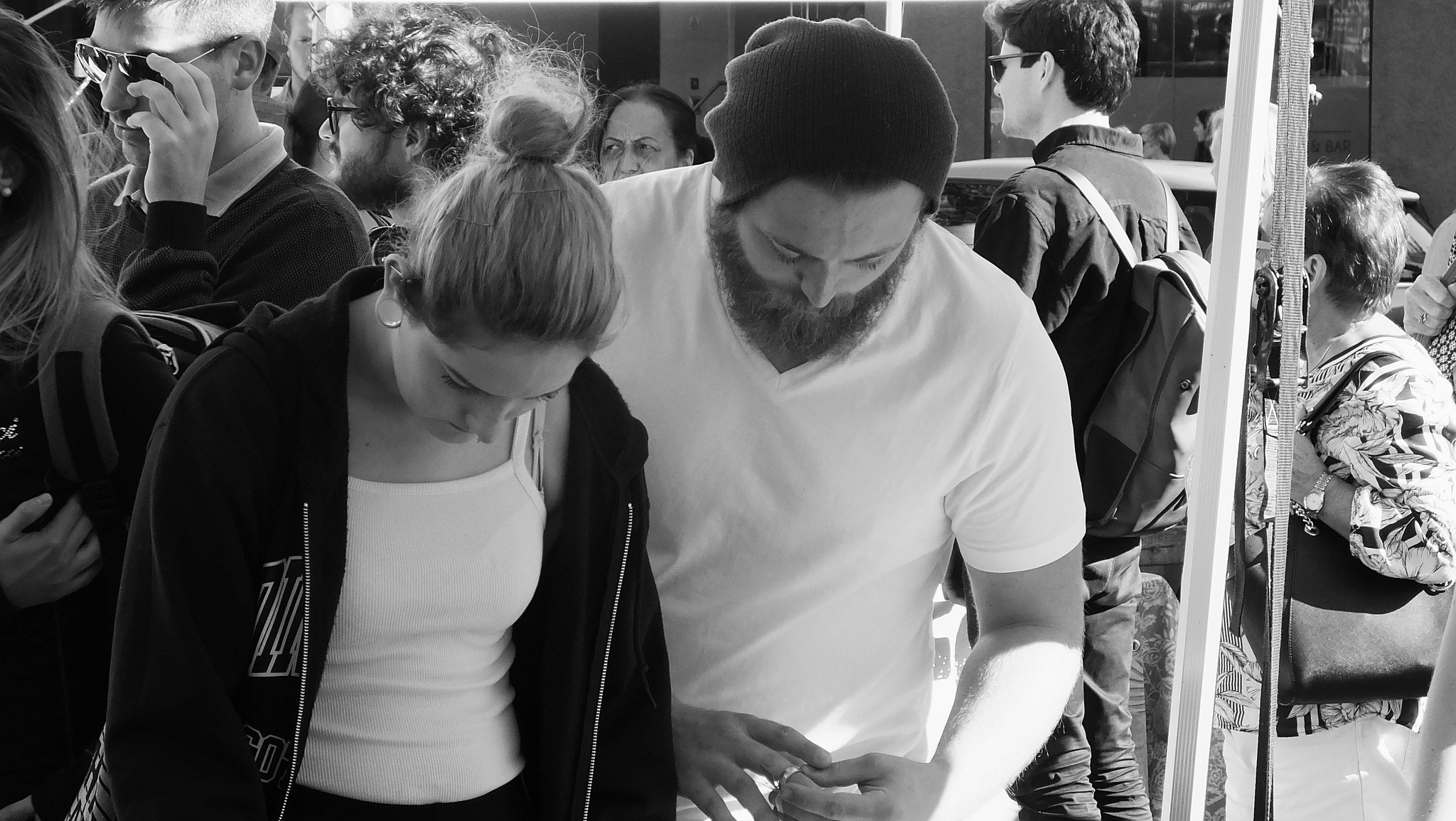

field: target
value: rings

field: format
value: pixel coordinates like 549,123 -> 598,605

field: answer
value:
778,765 -> 799,793
1418,312 -> 1427,324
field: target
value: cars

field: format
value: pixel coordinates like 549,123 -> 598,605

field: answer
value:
923,157 -> 1444,309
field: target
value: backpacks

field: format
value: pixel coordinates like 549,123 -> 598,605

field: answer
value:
1029,157 -> 1213,541
37,296 -> 232,568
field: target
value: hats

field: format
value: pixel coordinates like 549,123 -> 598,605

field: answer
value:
701,19 -> 956,217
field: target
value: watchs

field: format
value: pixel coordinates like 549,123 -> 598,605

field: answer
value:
1302,470 -> 1335,519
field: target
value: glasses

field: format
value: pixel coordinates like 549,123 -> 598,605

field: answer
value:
987,48 -> 1073,86
72,34 -> 246,93
327,92 -> 372,133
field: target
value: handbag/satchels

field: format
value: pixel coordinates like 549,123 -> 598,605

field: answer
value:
1278,349 -> 1454,693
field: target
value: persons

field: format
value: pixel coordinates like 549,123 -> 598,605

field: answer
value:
78,22 -> 678,820
1138,121 -> 1176,163
975,0 -> 1204,821
0,0 -> 700,821
1187,92 -> 1456,821
573,20 -> 1085,821
